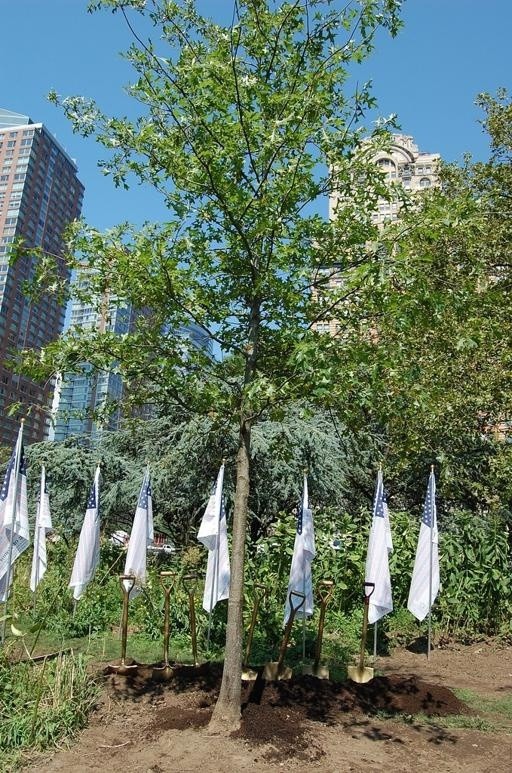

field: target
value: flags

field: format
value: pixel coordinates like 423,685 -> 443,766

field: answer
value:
282,476 -> 318,628
363,470 -> 395,625
196,465 -> 230,614
67,467 -> 101,601
406,472 -> 441,622
122,465 -> 155,601
0,424 -> 30,603
30,466 -> 53,593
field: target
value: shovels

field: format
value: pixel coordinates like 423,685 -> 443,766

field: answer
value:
108,571 -> 374,684
